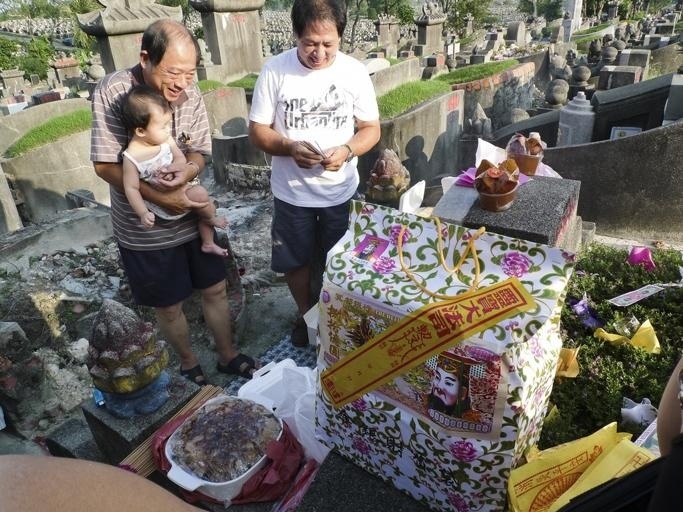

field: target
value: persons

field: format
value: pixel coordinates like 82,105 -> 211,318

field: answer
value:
90,18 -> 267,388
421,350 -> 479,424
248,2 -> 382,347
122,82 -> 229,257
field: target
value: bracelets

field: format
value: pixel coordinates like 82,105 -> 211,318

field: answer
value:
188,161 -> 201,176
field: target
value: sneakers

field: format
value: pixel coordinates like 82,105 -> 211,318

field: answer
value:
292,322 -> 309,347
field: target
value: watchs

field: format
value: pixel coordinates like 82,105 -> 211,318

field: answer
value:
343,144 -> 353,163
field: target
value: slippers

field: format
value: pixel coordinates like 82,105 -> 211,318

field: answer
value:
180,365 -> 207,386
216,353 -> 259,379
